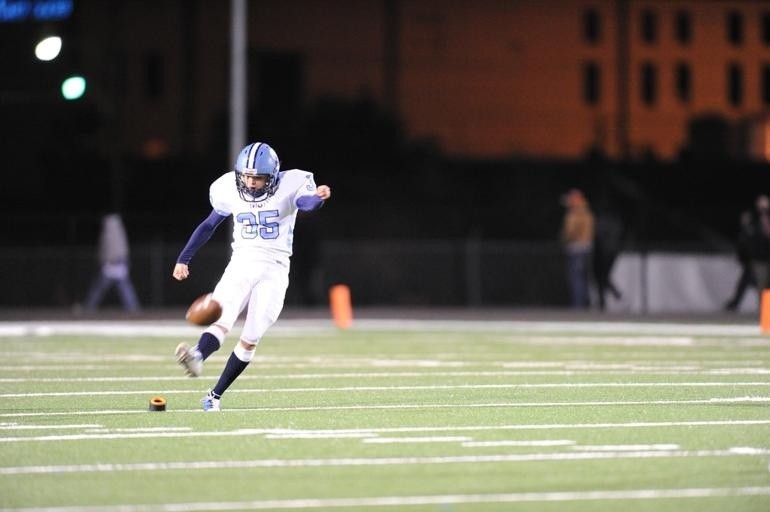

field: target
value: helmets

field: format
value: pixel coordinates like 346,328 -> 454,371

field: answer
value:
234,142 -> 280,183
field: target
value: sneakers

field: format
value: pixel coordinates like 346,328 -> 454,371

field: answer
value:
202,391 -> 221,411
175,342 -> 203,377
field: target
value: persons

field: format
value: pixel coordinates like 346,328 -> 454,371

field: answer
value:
70,215 -> 143,312
725,194 -> 770,310
560,186 -> 622,309
172,142 -> 331,412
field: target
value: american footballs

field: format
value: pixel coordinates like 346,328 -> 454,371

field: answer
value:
186,292 -> 222,326
